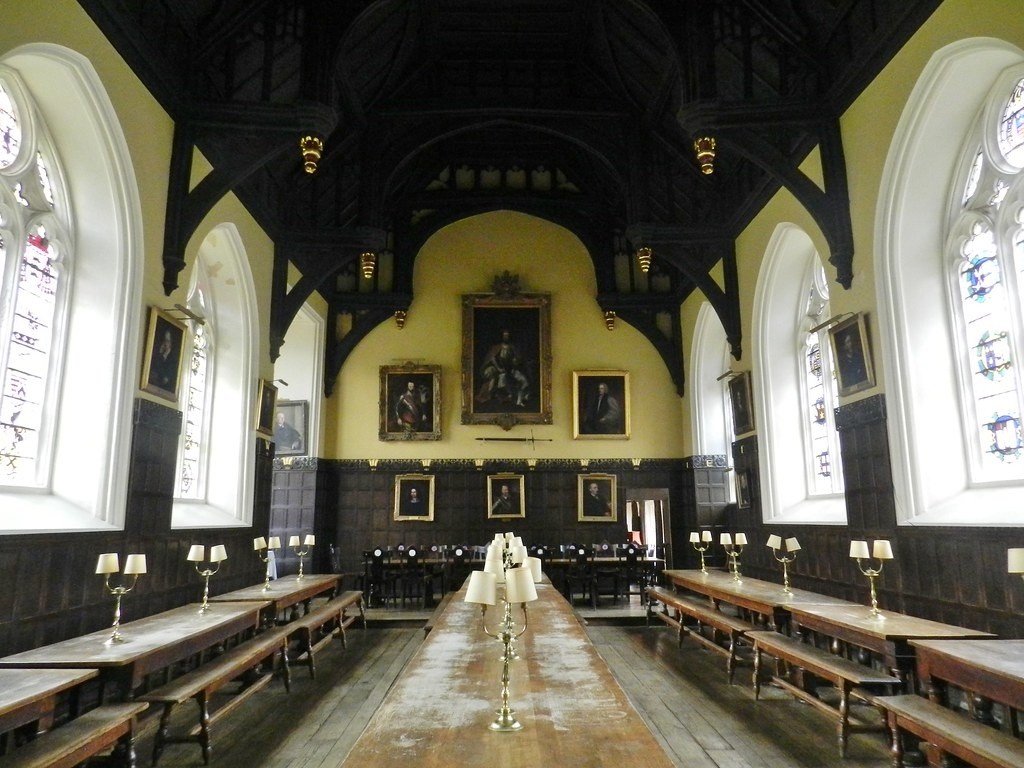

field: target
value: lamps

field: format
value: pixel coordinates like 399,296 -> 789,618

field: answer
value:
689,531 -> 1024,616
604,307 -> 616,330
636,244 -> 652,272
464,531 -> 542,732
394,307 -> 407,329
693,135 -> 716,174
300,130 -> 325,173
94,534 -> 317,645
360,247 -> 375,278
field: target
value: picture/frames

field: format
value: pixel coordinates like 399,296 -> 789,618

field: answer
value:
809,311 -> 875,397
140,303 -> 205,402
274,399 -> 309,455
379,270 -> 632,520
717,369 -> 755,436
256,377 -> 288,436
736,467 -> 752,509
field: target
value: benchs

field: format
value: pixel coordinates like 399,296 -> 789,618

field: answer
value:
0,591 -> 363,768
642,586 -> 1024,768
423,591 -> 456,640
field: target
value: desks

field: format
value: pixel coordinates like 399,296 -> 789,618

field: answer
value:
0,573 -> 345,760
662,569 -> 1024,768
340,572 -> 678,767
355,557 -> 666,605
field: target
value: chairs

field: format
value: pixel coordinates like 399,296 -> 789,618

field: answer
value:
363,538 -> 662,611
